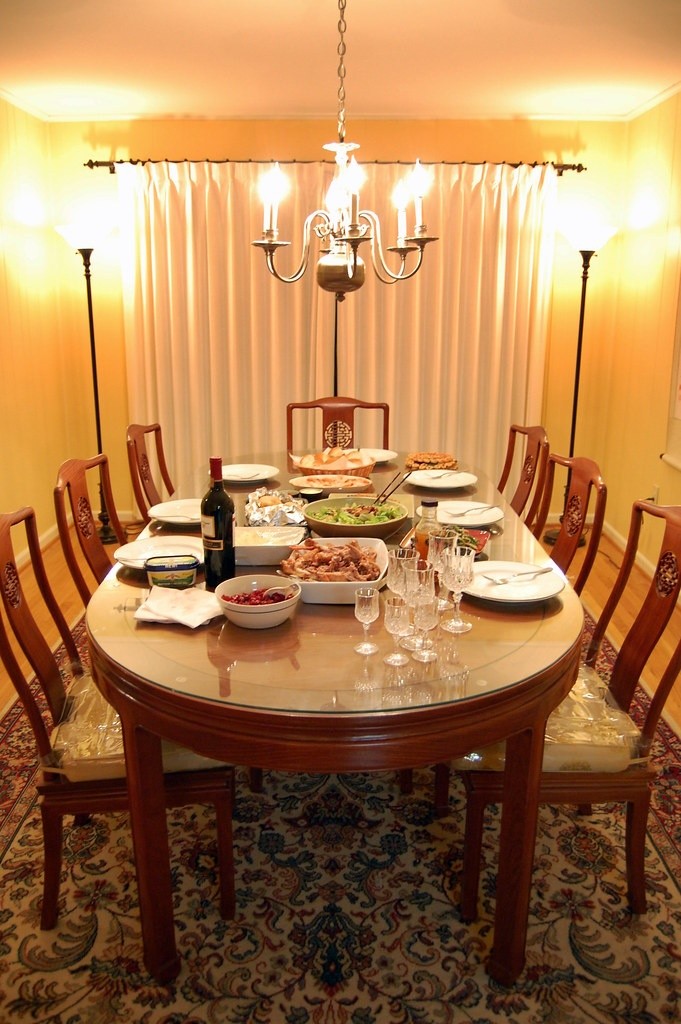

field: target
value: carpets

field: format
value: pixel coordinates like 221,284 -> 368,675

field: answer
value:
0,603 -> 681,1024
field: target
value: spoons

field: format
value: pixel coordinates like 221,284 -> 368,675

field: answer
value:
264,584 -> 299,599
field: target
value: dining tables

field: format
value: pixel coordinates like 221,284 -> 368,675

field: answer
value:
84,447 -> 584,989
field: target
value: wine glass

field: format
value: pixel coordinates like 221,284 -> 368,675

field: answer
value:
354,589 -> 380,655
383,549 -> 441,666
428,530 -> 477,634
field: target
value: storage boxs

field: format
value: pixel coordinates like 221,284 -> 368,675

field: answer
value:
143,555 -> 199,588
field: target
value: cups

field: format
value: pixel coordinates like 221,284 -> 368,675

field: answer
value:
300,488 -> 324,502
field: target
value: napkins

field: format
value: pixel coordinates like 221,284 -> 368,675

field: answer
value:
132,585 -> 224,629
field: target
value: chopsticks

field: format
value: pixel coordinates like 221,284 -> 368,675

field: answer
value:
374,472 -> 411,505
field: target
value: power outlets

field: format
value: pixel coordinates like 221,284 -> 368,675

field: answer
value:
652,484 -> 659,504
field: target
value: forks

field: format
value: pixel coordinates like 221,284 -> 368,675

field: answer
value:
425,470 -> 468,479
224,474 -> 260,479
482,568 -> 553,584
148,514 -> 200,520
446,504 -> 502,517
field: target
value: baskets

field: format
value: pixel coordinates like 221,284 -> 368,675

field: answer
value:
293,459 -> 375,478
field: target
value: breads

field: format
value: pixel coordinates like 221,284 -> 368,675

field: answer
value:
258,495 -> 281,507
300,446 -> 364,469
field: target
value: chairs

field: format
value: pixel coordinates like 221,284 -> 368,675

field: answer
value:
1,395 -> 681,931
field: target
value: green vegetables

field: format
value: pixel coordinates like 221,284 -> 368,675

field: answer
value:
441,525 -> 479,552
307,502 -> 404,524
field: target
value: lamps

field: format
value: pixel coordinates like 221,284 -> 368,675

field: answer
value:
251,0 -> 439,303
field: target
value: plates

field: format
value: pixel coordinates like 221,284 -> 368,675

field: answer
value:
343,448 -> 398,464
460,560 -> 566,602
147,499 -> 203,525
416,501 -> 504,528
114,535 -> 205,571
289,474 -> 372,495
329,493 -> 414,519
404,470 -> 478,489
208,463 -> 279,483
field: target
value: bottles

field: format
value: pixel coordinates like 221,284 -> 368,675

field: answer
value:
415,499 -> 443,593
200,457 -> 236,588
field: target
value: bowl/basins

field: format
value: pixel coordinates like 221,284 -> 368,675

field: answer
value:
301,497 -> 409,539
235,527 -> 305,565
459,529 -> 491,556
215,575 -> 302,629
286,537 -> 389,604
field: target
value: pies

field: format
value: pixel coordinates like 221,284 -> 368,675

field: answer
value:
407,453 -> 455,470
307,476 -> 368,486
